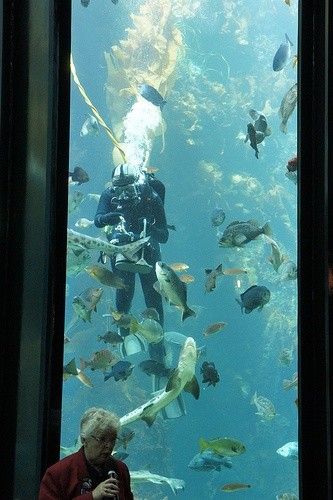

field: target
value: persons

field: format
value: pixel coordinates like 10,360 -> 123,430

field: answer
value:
38,407 -> 135,500
94,164 -> 187,377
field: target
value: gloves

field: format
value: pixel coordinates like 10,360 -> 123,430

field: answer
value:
101,211 -> 122,227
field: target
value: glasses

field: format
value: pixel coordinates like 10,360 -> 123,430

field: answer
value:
91,435 -> 120,444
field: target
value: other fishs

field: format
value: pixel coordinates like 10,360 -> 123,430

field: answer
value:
61,1 -> 298,496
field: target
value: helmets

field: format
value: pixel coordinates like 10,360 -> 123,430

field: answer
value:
112,163 -> 140,200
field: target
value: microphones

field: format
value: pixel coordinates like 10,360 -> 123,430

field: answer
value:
108,470 -> 119,500
